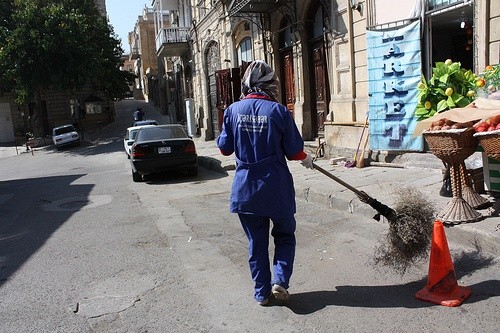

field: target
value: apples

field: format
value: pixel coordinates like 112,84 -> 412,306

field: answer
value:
476,115 -> 500,132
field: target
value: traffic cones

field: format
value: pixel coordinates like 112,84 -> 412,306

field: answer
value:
416,220 -> 471,308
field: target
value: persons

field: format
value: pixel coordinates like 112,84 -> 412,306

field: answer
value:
216,60 -> 314,306
133,107 -> 145,122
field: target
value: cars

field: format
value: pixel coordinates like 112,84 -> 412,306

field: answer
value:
133,119 -> 160,126
52,123 -> 80,149
130,124 -> 198,182
123,125 -> 157,159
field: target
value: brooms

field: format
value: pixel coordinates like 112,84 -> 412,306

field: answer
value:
312,162 -> 438,281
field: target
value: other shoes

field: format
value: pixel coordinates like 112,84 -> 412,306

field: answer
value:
271,284 -> 290,302
258,292 -> 272,306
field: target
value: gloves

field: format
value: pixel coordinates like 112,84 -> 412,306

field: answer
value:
301,154 -> 313,170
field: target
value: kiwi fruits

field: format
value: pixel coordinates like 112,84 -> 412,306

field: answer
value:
426,118 -> 476,133
